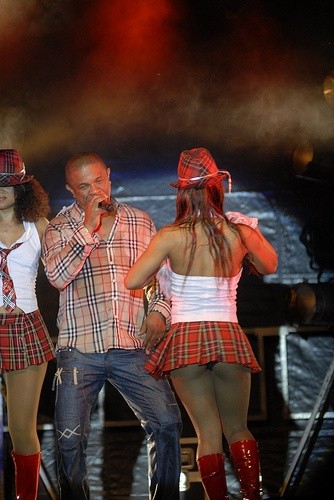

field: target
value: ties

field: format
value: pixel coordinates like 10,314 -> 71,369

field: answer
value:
0,242 -> 25,314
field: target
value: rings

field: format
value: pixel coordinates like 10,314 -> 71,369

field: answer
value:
155,333 -> 163,343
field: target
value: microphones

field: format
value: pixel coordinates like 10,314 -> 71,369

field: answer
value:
98,201 -> 114,212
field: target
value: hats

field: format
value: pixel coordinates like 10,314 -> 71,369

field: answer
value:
168,148 -> 228,191
0,149 -> 35,187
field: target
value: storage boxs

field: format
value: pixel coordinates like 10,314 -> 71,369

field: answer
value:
2,326 -> 334,432
179,438 -> 209,500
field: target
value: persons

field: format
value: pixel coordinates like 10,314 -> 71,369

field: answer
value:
40,148 -> 183,500
125,146 -> 278,500
1,146 -> 52,499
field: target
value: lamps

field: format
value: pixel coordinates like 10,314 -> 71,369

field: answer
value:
291,138 -> 334,183
289,282 -> 333,324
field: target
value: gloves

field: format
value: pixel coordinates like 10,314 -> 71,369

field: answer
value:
223,211 -> 258,231
